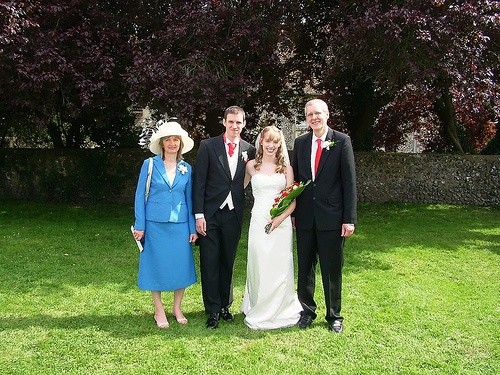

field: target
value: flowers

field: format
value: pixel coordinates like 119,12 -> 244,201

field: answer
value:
322,139 -> 339,152
178,165 -> 189,175
265,180 -> 310,234
241,150 -> 250,164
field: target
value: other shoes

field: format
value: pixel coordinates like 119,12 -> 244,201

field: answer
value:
171,310 -> 188,324
154,313 -> 170,328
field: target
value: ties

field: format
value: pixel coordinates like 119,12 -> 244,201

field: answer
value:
223,132 -> 236,157
315,139 -> 322,176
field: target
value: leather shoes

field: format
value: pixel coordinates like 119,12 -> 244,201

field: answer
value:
329,320 -> 343,333
218,307 -> 234,321
296,314 -> 313,328
205,312 -> 220,329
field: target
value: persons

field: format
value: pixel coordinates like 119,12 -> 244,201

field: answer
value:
291,98 -> 356,333
134,122 -> 198,326
244,126 -> 303,329
193,106 -> 257,328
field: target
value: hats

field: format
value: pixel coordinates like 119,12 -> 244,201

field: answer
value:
149,122 -> 195,155
